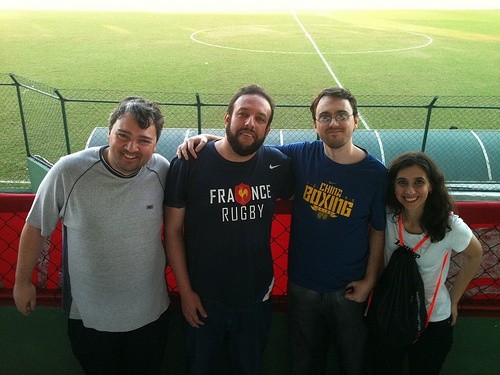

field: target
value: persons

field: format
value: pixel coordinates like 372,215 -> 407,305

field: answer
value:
358,152 -> 483,375
162,84 -> 295,375
13,97 -> 171,375
176,87 -> 390,375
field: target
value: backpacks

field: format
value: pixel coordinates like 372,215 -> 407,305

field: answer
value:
367,212 -> 453,348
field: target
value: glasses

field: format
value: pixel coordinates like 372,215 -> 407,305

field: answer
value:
315,110 -> 356,124
